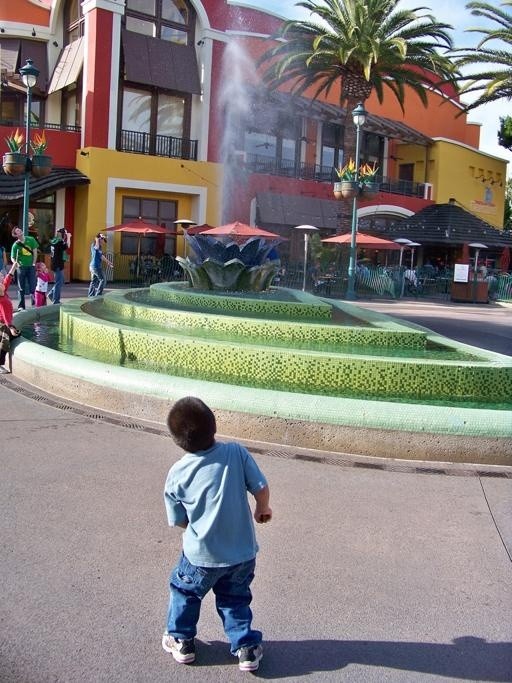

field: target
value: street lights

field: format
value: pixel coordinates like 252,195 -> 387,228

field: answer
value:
337,100 -> 370,299
19,57 -> 39,234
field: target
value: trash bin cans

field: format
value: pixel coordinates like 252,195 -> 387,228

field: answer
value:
451,280 -> 489,304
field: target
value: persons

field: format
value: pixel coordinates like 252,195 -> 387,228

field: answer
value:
89,233 -> 114,297
162,397 -> 272,670
35,262 -> 53,307
10,226 -> 39,309
47,229 -> 72,304
0,246 -> 7,270
0,263 -> 19,373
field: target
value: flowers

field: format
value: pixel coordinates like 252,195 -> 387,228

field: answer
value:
28,129 -> 48,154
328,157 -> 381,199
2,127 -> 28,152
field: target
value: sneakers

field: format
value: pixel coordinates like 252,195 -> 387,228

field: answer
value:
237,643 -> 265,674
161,627 -> 198,665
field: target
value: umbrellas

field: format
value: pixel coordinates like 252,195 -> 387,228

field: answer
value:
173,224 -> 215,235
101,218 -> 175,276
320,232 -> 405,274
199,221 -> 278,237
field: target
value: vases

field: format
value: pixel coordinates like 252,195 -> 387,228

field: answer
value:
2,151 -> 26,175
29,155 -> 53,179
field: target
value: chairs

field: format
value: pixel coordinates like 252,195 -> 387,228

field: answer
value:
128,252 -> 187,288
273,256 -> 510,300
